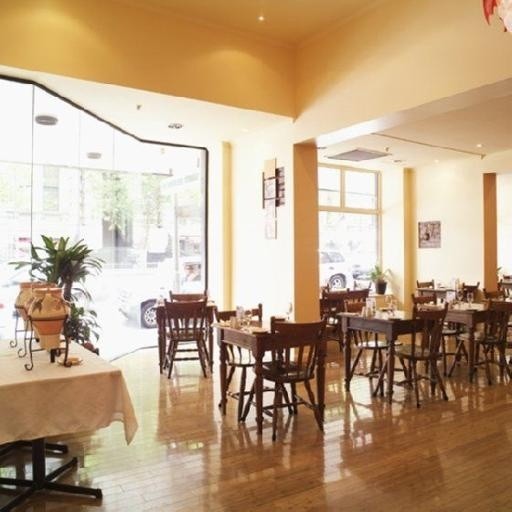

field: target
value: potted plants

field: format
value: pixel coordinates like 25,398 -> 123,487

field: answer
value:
10,234 -> 108,371
371,266 -> 394,294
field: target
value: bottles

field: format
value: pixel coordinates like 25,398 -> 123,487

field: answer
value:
455,279 -> 460,289
368,288 -> 376,317
230,316 -> 236,328
362,306 -> 366,319
233,317 -> 240,329
367,308 -> 371,320
244,311 -> 252,327
366,298 -> 372,317
237,305 -> 243,327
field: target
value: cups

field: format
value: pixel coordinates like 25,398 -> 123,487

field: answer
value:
447,291 -> 455,303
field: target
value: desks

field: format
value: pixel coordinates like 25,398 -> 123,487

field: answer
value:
1,334 -> 123,512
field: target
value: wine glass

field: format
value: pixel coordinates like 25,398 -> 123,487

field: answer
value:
390,300 -> 398,317
467,292 -> 474,308
384,296 -> 392,317
456,289 -> 464,309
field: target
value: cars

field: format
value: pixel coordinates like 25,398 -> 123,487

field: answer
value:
1,260 -> 88,337
121,246 -> 356,329
